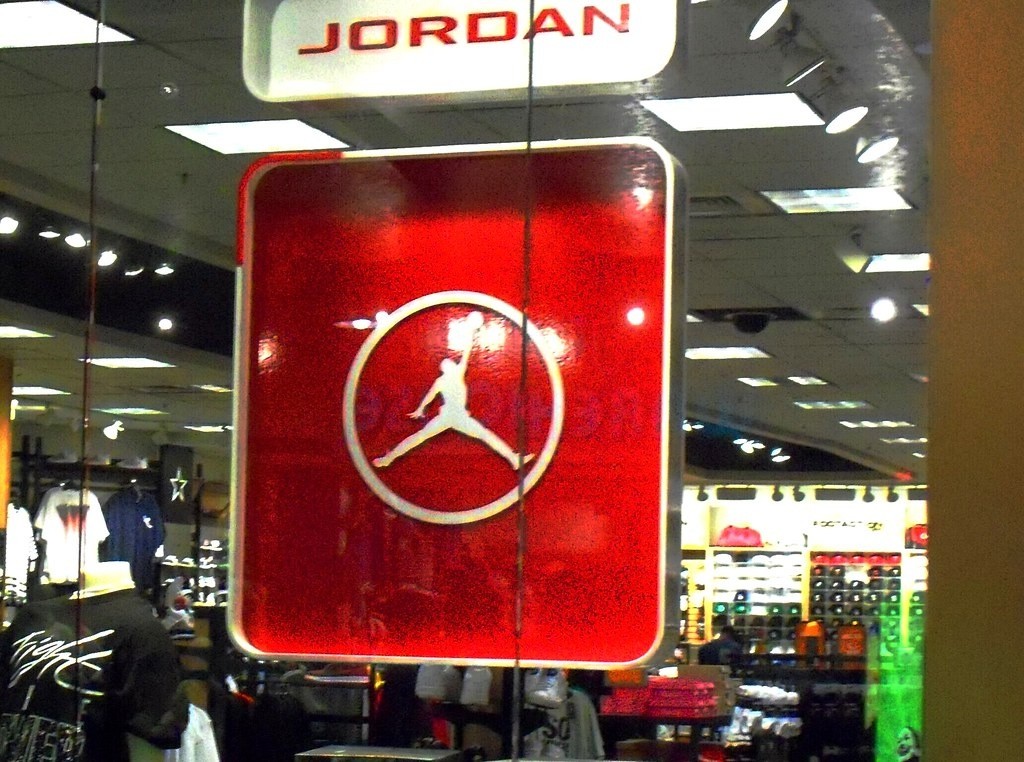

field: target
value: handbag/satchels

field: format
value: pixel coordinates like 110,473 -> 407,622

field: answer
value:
906,524 -> 927,548
716,525 -> 765,546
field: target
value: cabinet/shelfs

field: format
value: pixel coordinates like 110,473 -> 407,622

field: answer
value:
657,545 -> 929,740
160,545 -> 227,607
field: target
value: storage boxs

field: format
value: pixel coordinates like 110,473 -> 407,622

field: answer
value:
599,664 -> 742,717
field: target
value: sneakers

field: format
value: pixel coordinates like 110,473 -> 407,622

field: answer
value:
416,664 -> 462,699
459,667 -> 493,705
525,668 -> 568,707
153,607 -> 196,639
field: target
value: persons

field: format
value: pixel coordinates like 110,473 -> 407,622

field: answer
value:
697,625 -> 739,666
0,560 -> 189,762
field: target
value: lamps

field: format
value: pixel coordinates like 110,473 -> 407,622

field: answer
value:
38,223 -> 175,277
855,127 -> 899,163
823,75 -> 869,134
793,485 -> 805,503
697,485 -> 708,501
887,485 -> 899,502
863,486 -> 875,502
767,37 -> 829,87
722,0 -> 789,41
772,485 -> 784,501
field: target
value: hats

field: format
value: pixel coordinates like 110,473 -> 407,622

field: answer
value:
811,556 -> 901,641
714,589 -> 802,638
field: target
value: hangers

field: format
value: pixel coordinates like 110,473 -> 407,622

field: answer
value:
55,472 -> 81,490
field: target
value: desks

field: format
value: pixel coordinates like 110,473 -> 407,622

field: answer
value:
294,745 -> 461,762
